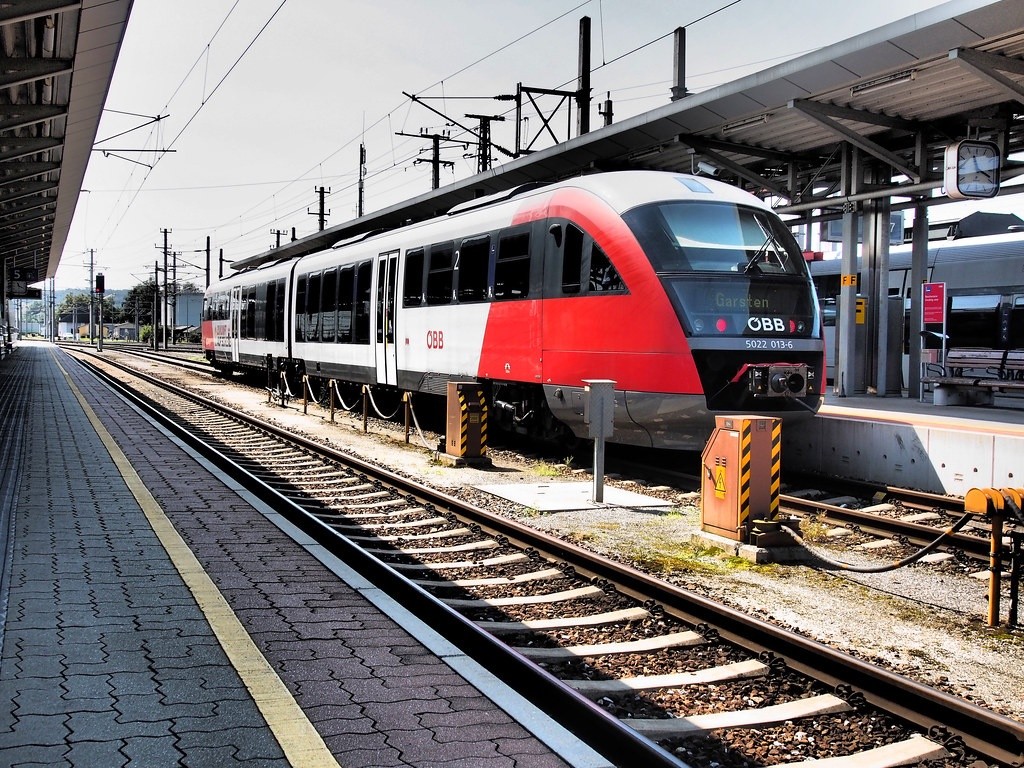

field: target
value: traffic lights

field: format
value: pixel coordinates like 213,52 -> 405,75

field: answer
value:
96,275 -> 104,293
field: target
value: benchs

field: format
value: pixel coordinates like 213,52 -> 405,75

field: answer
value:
881,349 -> 1024,364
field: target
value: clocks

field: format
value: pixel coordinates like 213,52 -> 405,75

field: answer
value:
944,139 -> 1000,200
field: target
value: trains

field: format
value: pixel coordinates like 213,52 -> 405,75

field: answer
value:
201,173 -> 830,451
804,210 -> 1024,412
